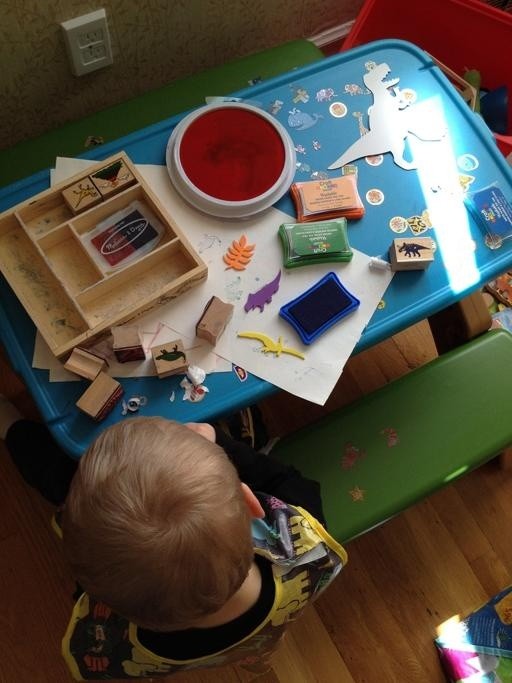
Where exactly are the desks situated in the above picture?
[1,40,512,473]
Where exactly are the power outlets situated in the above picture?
[61,9,115,78]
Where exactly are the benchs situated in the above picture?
[2,39,327,178]
[264,329,511,544]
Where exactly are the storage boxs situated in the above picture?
[334,1,512,157]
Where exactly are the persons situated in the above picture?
[0,385,350,681]
